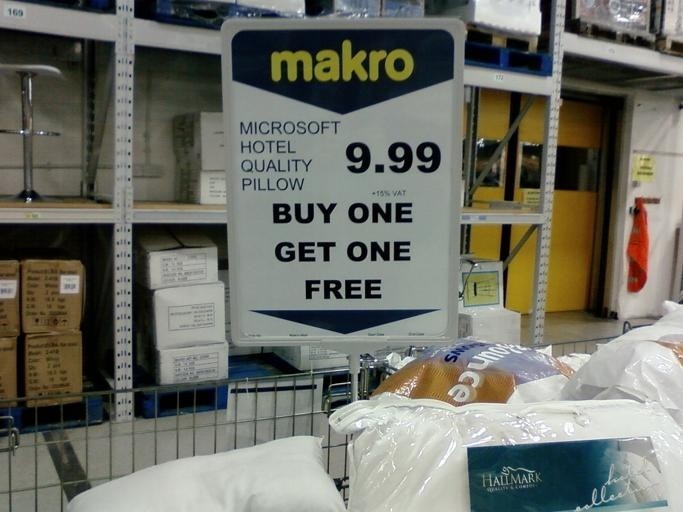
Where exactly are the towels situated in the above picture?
[628,197,651,290]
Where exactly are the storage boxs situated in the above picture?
[1,257,83,411]
[168,111,227,206]
[134,228,230,384]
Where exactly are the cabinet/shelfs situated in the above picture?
[0,0,571,433]
